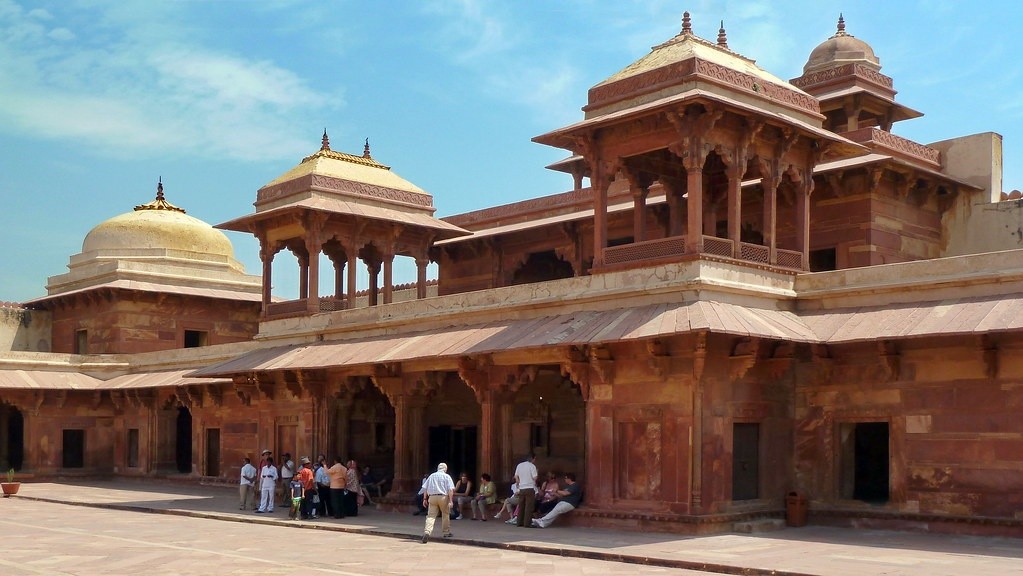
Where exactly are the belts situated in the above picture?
[284,478,288,479]
[433,494,442,495]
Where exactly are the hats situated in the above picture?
[301,458,310,464]
[262,449,272,456]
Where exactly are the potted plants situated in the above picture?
[1,468,21,494]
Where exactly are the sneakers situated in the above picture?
[532,518,544,528]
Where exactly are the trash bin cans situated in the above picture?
[786,488,807,527]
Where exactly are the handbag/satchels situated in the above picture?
[313,489,320,503]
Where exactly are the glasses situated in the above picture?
[282,458,286,459]
[550,477,556,480]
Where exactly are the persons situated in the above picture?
[421,464,456,544]
[239,450,386,523]
[412,452,583,529]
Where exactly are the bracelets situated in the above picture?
[481,494,483,496]
[548,500,549,502]
[424,499,427,501]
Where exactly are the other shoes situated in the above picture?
[239,508,243,510]
[456,514,464,519]
[255,511,264,513]
[279,505,291,507]
[509,517,518,524]
[288,517,292,519]
[471,518,478,520]
[308,513,313,519]
[269,510,273,513]
[505,519,514,523]
[301,514,307,519]
[295,518,299,520]
[494,513,501,518]
[443,533,453,537]
[422,533,429,543]
[480,518,487,521]
